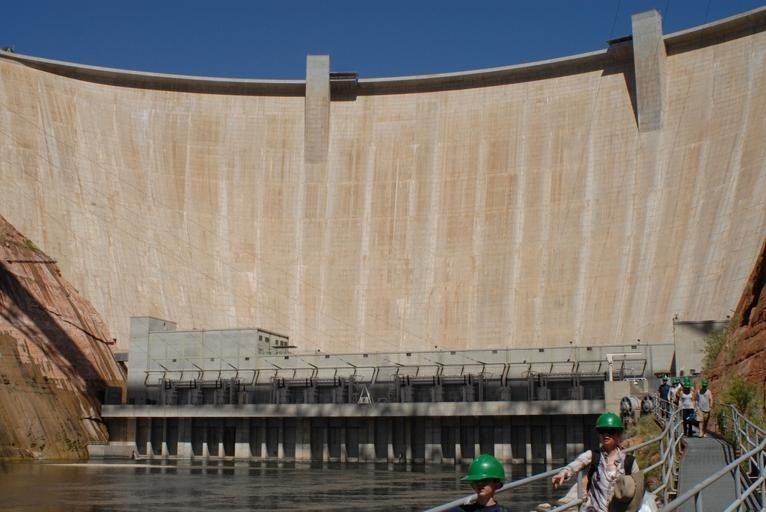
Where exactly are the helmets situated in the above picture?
[662,374,708,389]
[597,411,626,431]
[460,455,505,488]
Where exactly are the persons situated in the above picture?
[660,376,713,437]
[450,454,513,512]
[551,412,639,512]
